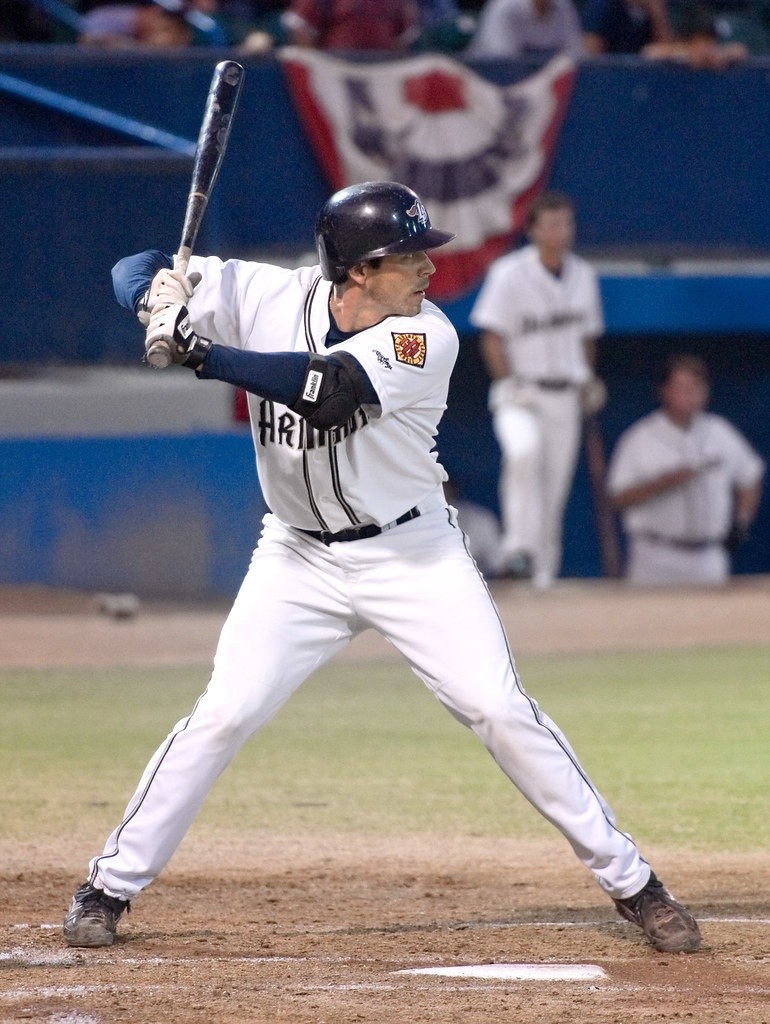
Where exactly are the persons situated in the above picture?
[603,354,764,583]
[65,181,702,954]
[467,193,605,583]
[466,0,746,69]
[77,0,228,47]
[287,0,420,50]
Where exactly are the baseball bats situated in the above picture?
[146,60,246,369]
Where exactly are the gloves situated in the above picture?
[138,269,213,377]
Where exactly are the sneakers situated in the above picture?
[611,869,704,951]
[63,881,127,948]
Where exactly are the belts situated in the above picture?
[301,506,420,548]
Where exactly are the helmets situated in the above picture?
[314,180,457,281]
